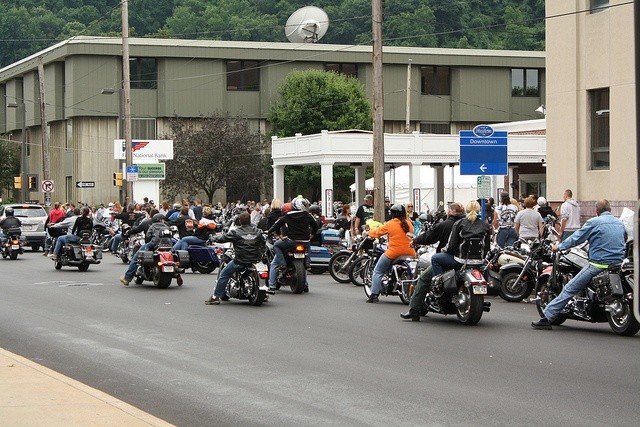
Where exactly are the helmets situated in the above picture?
[309,204,320,212]
[153,213,166,222]
[290,195,311,210]
[4,208,14,217]
[281,203,293,213]
[390,204,406,219]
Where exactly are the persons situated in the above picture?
[169,205,180,221]
[559,189,580,239]
[49,202,65,246]
[48,209,83,260]
[446,201,455,207]
[66,203,75,216]
[97,204,106,222]
[144,197,148,203]
[111,202,124,221]
[252,201,255,211]
[401,203,466,321]
[510,198,524,210]
[159,202,171,217]
[274,198,318,279]
[226,202,235,221]
[103,202,114,225]
[166,204,180,218]
[517,193,528,209]
[181,199,196,220]
[263,199,269,208]
[236,200,240,208]
[173,207,216,251]
[110,204,134,253]
[365,204,416,303]
[265,203,292,293]
[431,200,490,293]
[135,203,141,210]
[205,212,266,305]
[247,201,251,209]
[209,203,213,208]
[78,201,82,207]
[62,208,94,256]
[491,192,518,248]
[149,200,156,208]
[268,198,283,227]
[515,197,545,253]
[385,196,391,215]
[256,202,263,214]
[257,209,270,230]
[310,205,323,246]
[144,213,172,250]
[43,216,53,257]
[0,206,23,250]
[531,198,628,329]
[61,204,65,209]
[144,204,150,210]
[2,208,22,255]
[65,203,70,209]
[216,202,223,208]
[119,209,159,286]
[486,197,494,224]
[406,204,419,221]
[355,195,374,235]
[537,197,557,219]
[167,207,194,240]
[81,203,84,212]
[335,205,352,230]
[195,200,203,220]
[134,211,141,253]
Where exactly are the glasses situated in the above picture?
[385,200,390,203]
[408,206,413,207]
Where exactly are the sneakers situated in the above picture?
[48,253,57,259]
[205,295,220,305]
[120,278,129,286]
[177,273,182,286]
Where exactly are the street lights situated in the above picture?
[7,102,25,203]
[102,88,127,206]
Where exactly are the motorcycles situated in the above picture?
[91,218,131,263]
[48,223,103,271]
[535,234,640,337]
[364,234,419,304]
[0,227,24,259]
[124,230,189,289]
[406,232,491,325]
[263,231,309,294]
[308,229,375,287]
[207,234,275,306]
[487,202,564,302]
[171,233,223,273]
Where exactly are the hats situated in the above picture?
[537,197,546,205]
[364,195,373,200]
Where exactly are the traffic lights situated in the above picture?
[12,175,22,189]
[113,173,123,186]
[28,176,37,189]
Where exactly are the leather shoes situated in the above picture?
[531,317,552,330]
[400,308,420,321]
[367,295,378,303]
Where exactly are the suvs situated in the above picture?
[1,201,51,253]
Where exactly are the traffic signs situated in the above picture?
[76,181,95,187]
[460,125,508,175]
